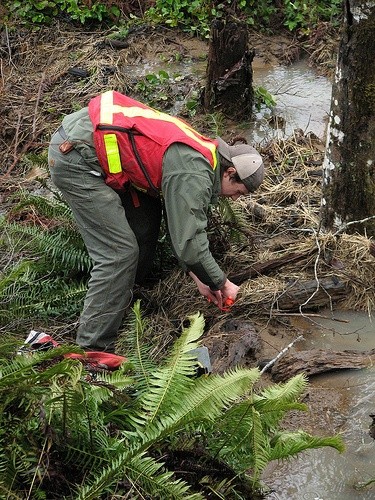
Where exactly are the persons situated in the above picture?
[47,89,265,354]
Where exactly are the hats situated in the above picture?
[215,134,265,192]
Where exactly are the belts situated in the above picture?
[58,126,67,140]
